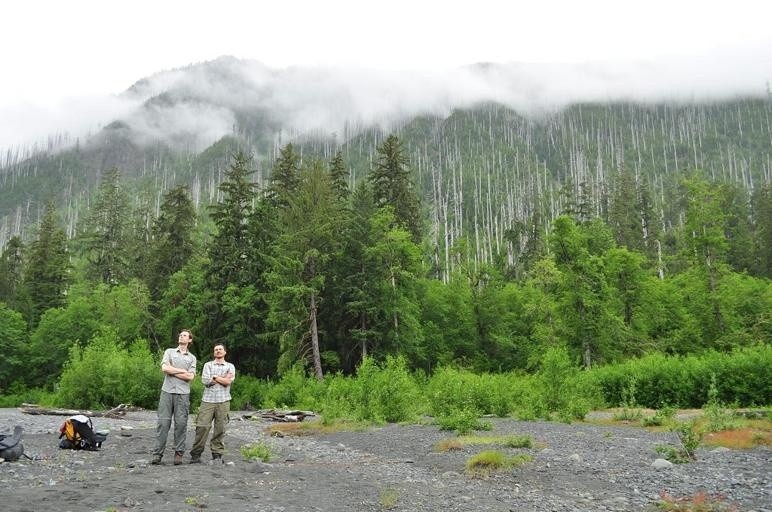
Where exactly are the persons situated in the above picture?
[189,341,235,464]
[153,327,197,465]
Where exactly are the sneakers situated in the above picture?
[173,452,183,465]
[213,455,224,463]
[152,453,163,464]
[190,455,200,464]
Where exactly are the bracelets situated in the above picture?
[213,374,217,380]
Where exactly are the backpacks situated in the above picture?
[0,427,33,463]
